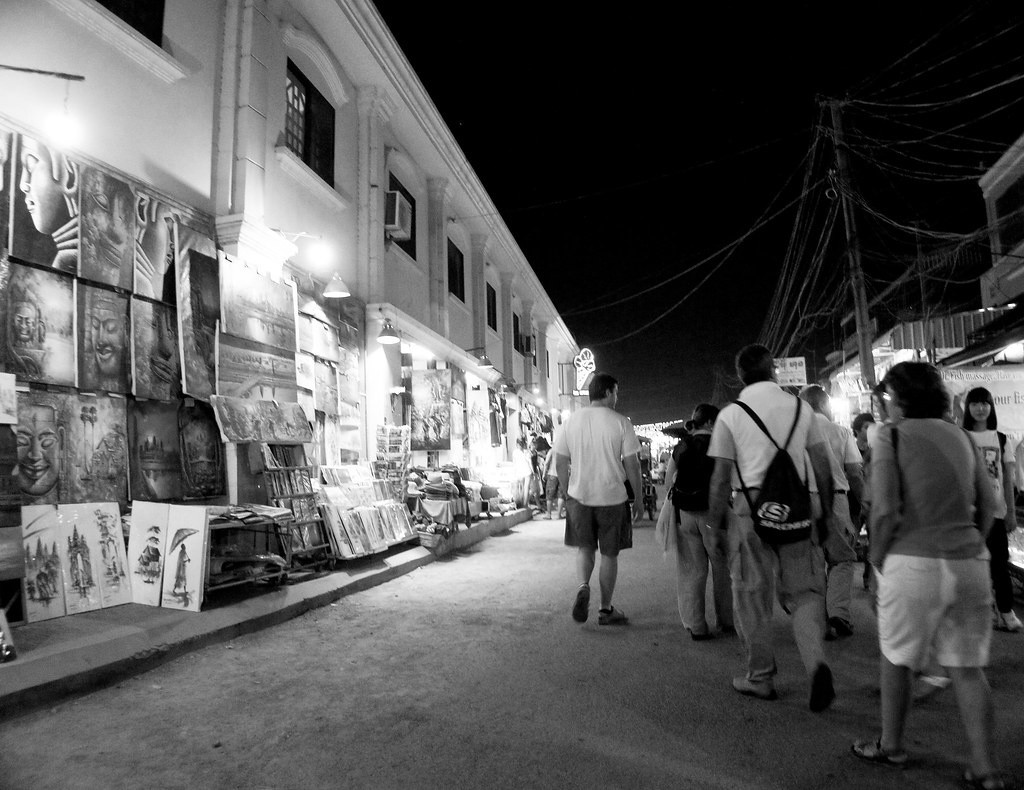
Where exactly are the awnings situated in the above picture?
[823,303,1024,380]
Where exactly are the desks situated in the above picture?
[417,497,469,535]
[204,518,294,601]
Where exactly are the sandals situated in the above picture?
[961,770,1008,790]
[850,740,913,770]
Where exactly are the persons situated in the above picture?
[647,458,670,483]
[850,361,1009,790]
[794,383,865,641]
[961,385,1024,632]
[846,413,877,592]
[510,439,567,523]
[665,401,737,642]
[703,343,836,713]
[554,372,646,626]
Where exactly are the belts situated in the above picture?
[834,489,847,493]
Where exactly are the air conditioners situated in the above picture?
[385,191,412,241]
[525,335,536,357]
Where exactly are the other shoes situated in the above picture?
[691,631,708,640]
[733,677,775,700]
[716,622,734,632]
[809,662,834,712]
[994,614,999,628]
[828,617,853,635]
[572,584,591,622]
[825,621,839,640]
[595,607,624,623]
[1001,610,1022,631]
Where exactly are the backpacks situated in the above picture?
[728,396,812,545]
[671,434,715,511]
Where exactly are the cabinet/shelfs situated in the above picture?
[373,423,411,506]
[259,443,336,576]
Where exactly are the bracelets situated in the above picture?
[867,553,881,566]
[705,524,718,536]
[1007,509,1017,513]
[634,498,644,504]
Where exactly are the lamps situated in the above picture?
[478,348,493,369]
[323,272,350,299]
[376,318,401,344]
